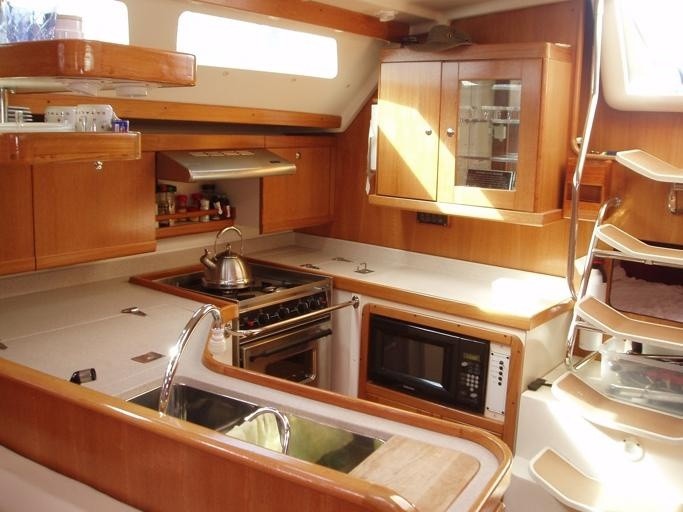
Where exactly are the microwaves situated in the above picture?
[365,311,491,418]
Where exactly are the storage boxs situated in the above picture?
[600,336,683,418]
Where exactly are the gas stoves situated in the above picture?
[154,261,320,304]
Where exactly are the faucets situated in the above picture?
[156,302,227,411]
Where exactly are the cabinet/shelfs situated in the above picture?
[157,150,297,249]
[0,39,196,167]
[260,148,334,234]
[563,197,682,419]
[377,60,543,214]
[0,145,38,274]
[31,146,157,272]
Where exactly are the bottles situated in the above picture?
[156,182,232,226]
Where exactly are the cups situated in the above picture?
[44,104,121,133]
[54,14,84,39]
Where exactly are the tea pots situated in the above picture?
[198,225,254,293]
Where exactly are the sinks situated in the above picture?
[223,404,391,476]
[114,374,260,431]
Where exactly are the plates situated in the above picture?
[1,122,77,133]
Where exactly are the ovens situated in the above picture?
[231,284,336,389]
